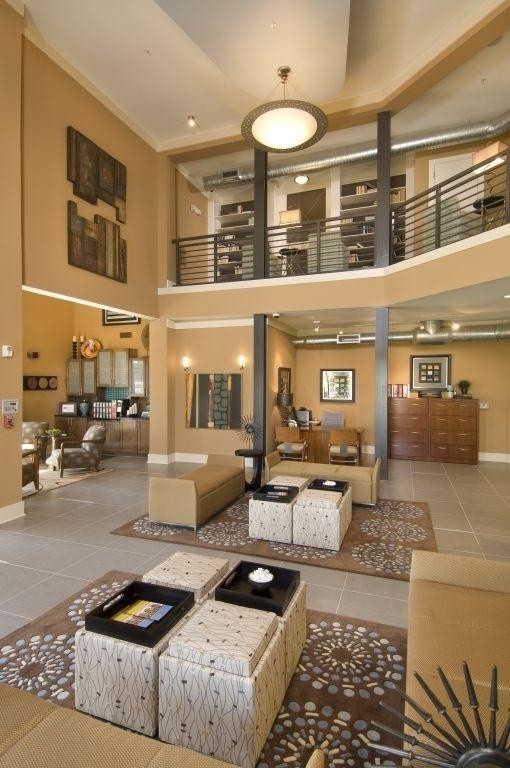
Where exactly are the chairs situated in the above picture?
[275,424,361,466]
[23,421,107,492]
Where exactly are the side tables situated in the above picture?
[235,448,265,490]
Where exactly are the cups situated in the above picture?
[447,385,454,392]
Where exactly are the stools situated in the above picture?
[280,249,300,276]
[474,197,505,230]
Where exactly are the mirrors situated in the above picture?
[184,372,243,433]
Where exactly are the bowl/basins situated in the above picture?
[244,572,275,591]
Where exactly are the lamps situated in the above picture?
[241,67,327,153]
[472,141,508,172]
[278,204,303,229]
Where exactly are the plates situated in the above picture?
[27,377,38,388]
[39,378,48,389]
[49,377,58,389]
[80,338,101,358]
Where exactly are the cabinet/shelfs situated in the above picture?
[217,251,242,280]
[53,414,149,456]
[347,246,374,266]
[428,396,479,466]
[386,397,429,465]
[65,347,149,397]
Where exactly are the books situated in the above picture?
[341,218,354,224]
[357,185,367,194]
[346,241,364,248]
[248,217,254,225]
[218,256,229,263]
[216,246,240,253]
[347,253,358,262]
[389,190,406,203]
[387,384,409,399]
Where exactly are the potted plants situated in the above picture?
[458,380,470,395]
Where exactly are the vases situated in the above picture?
[79,402,90,417]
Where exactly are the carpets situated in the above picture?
[1,566,407,768]
[22,463,114,499]
[110,493,437,581]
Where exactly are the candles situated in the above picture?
[80,335,85,342]
[72,335,77,342]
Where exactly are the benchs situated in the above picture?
[1,681,240,768]
[264,450,382,506]
[147,455,245,532]
[403,548,510,767]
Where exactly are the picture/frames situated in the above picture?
[319,368,355,403]
[102,309,141,326]
[409,354,451,390]
[278,367,291,394]
